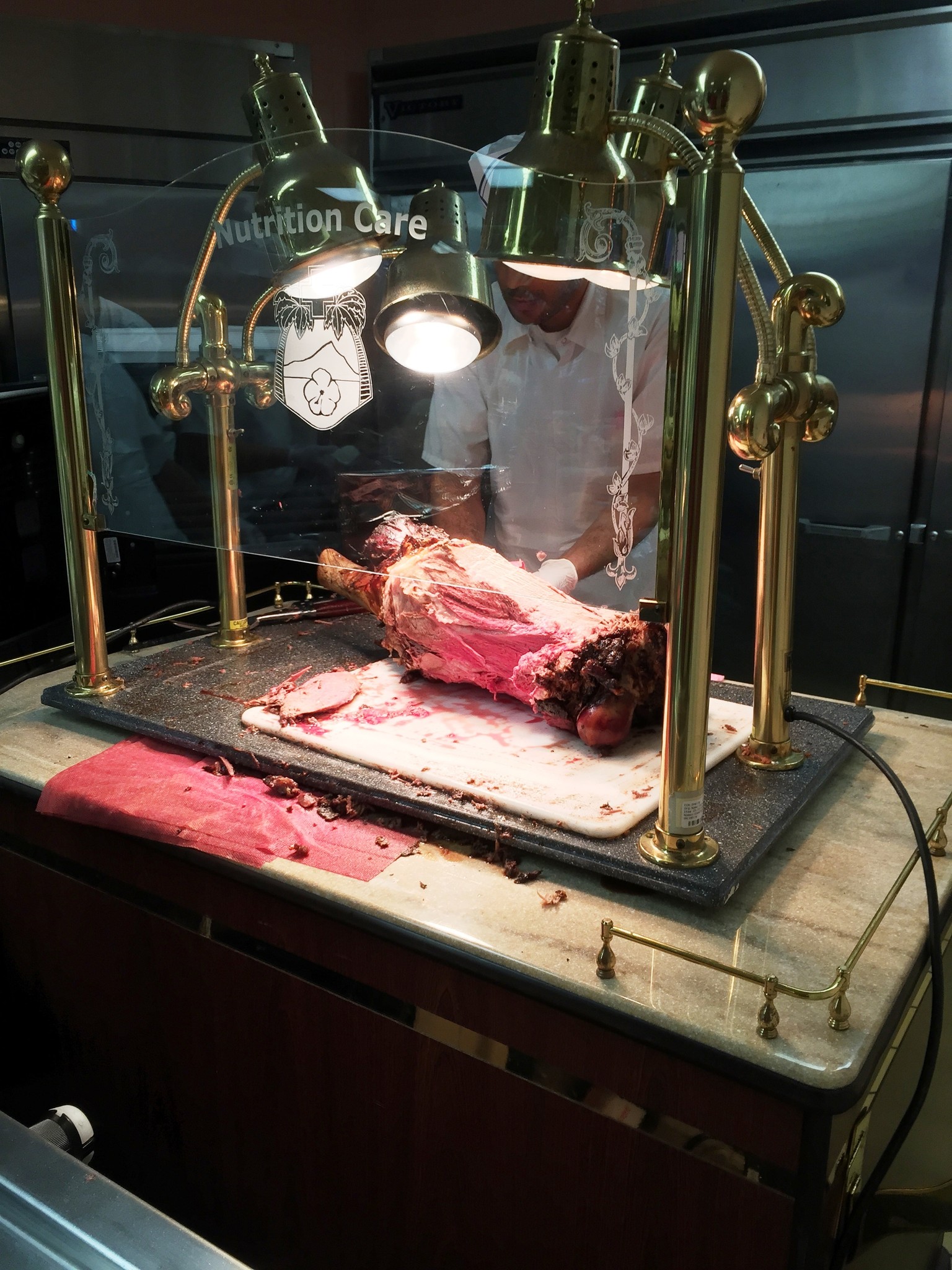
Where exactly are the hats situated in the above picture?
[467,131,526,219]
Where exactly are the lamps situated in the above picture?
[151,50,503,650]
[485,1,847,773]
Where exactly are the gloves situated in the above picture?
[531,558,579,593]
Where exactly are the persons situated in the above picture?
[423,136,669,610]
[48,278,181,547]
